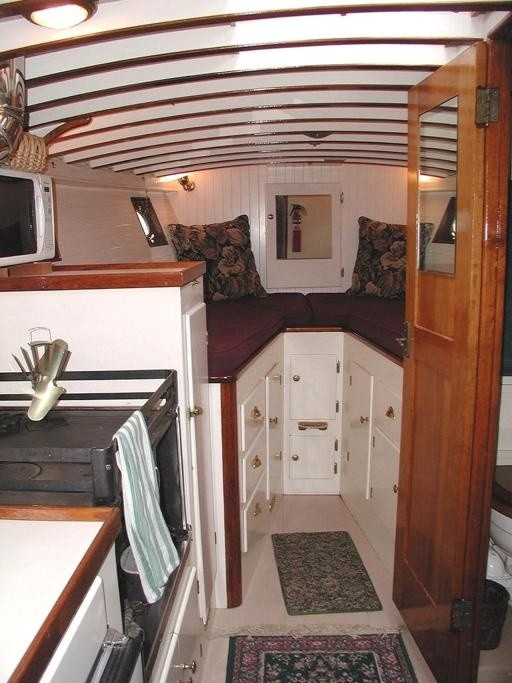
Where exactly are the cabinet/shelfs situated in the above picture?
[284,331,340,496]
[0,260,218,624]
[341,333,403,579]
[208,328,285,609]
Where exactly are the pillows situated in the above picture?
[345,214,435,304]
[170,214,267,302]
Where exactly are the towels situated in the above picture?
[112,410,182,604]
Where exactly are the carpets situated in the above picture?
[225,632,419,683]
[272,531,383,615]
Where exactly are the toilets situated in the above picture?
[486,375,512,609]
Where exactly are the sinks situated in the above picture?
[1,520,103,681]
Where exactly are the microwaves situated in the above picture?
[0,167,58,267]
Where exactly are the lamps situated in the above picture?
[18,0,98,30]
[177,174,195,192]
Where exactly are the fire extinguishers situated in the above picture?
[289,204,305,252]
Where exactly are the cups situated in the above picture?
[477,578,510,651]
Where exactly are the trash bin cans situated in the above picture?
[480,580,509,651]
